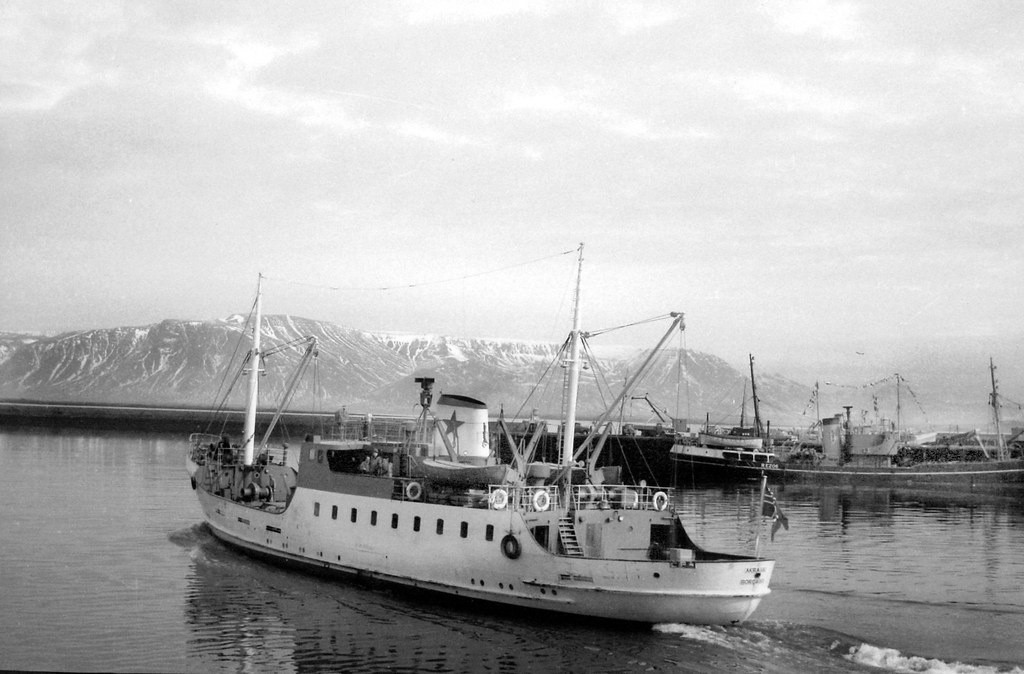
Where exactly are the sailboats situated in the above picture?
[184,242,776,626]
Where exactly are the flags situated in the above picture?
[761,485,788,542]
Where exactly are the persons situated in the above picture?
[358,448,393,477]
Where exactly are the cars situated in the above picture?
[558,422,590,435]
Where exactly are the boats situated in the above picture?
[669,427,779,470]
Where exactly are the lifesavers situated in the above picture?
[653,491,669,512]
[533,490,549,511]
[406,482,421,499]
[492,489,507,508]
[500,534,521,559]
